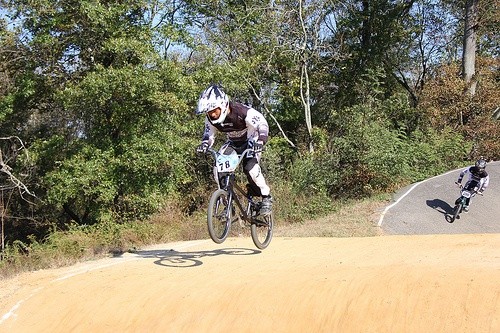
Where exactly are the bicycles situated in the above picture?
[451,180,484,225]
[193,144,275,250]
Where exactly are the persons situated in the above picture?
[191,84,273,218]
[456,158,489,213]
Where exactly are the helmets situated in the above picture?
[475,159,486,174]
[197,85,229,124]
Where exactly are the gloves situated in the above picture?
[457,181,461,187]
[252,139,264,152]
[197,142,209,154]
[479,188,483,194]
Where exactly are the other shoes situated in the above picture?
[259,194,274,217]
[464,205,469,211]
[220,205,235,218]
[455,197,462,204]
